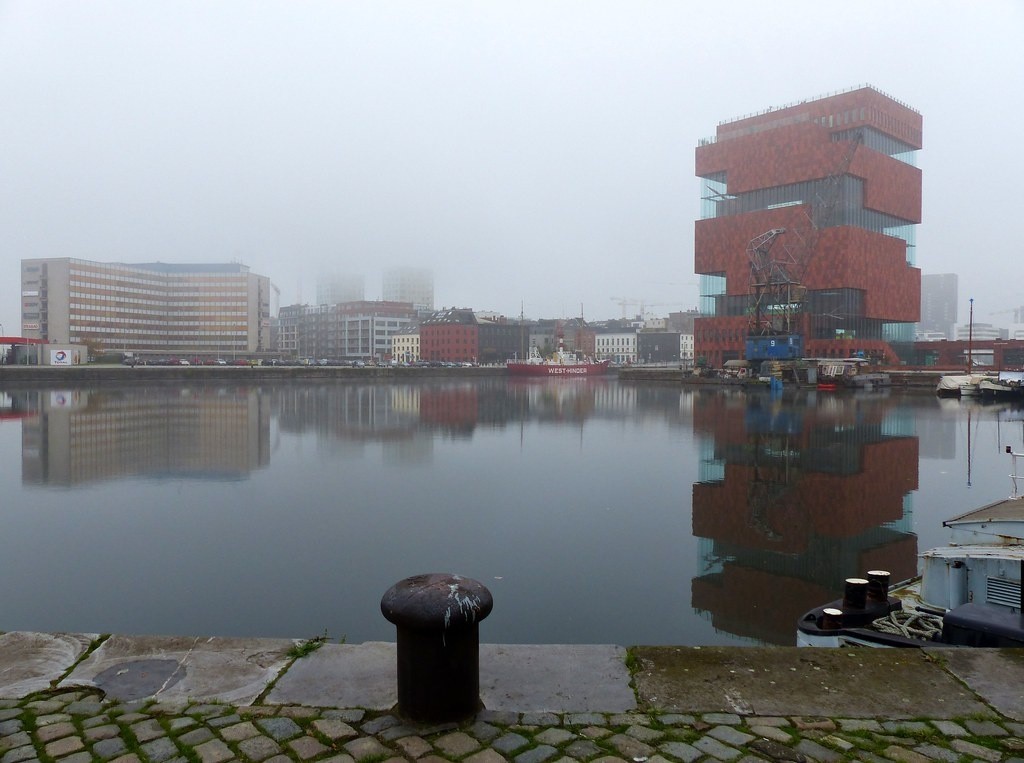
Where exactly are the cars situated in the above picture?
[122,358,480,368]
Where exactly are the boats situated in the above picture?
[505,317,611,377]
[842,368,893,388]
[795,445,1024,648]
[938,297,1024,399]
[684,367,748,386]
